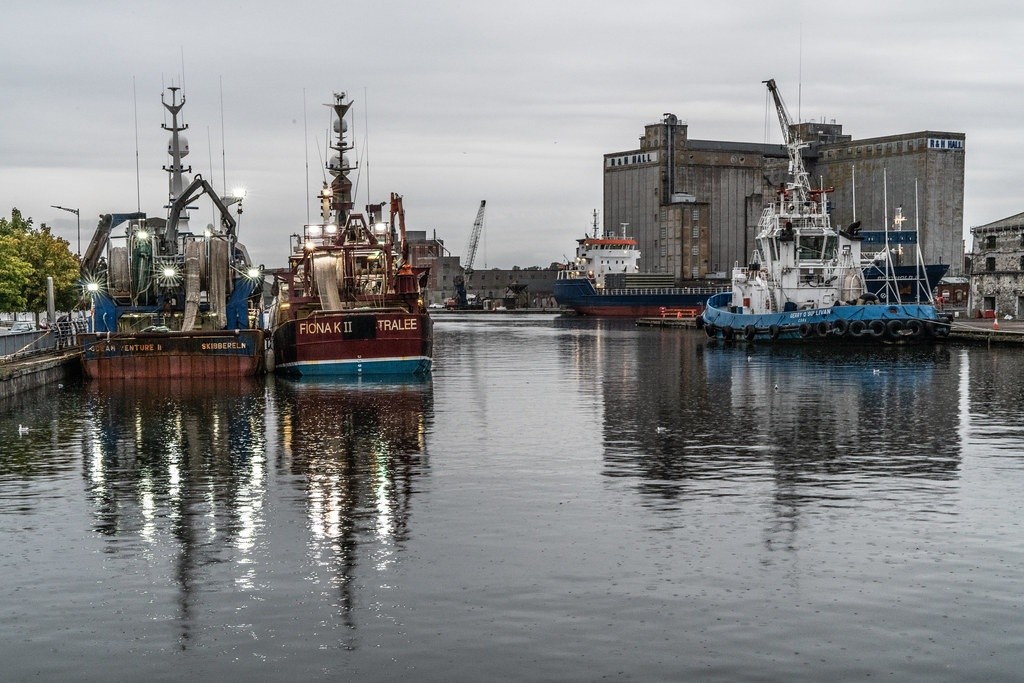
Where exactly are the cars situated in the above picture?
[39,317,47,329]
[8,322,33,333]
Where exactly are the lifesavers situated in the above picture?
[868,320,887,337]
[705,324,714,337]
[849,320,867,338]
[816,320,831,338]
[906,320,923,338]
[768,324,779,339]
[743,324,755,340]
[696,315,702,329]
[832,318,849,338]
[798,323,812,337]
[887,320,905,338]
[758,270,768,279]
[721,326,732,342]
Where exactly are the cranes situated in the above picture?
[445,199,487,310]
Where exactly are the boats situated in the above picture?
[555,208,732,316]
[695,78,952,344]
[80,48,272,380]
[265,86,434,378]
